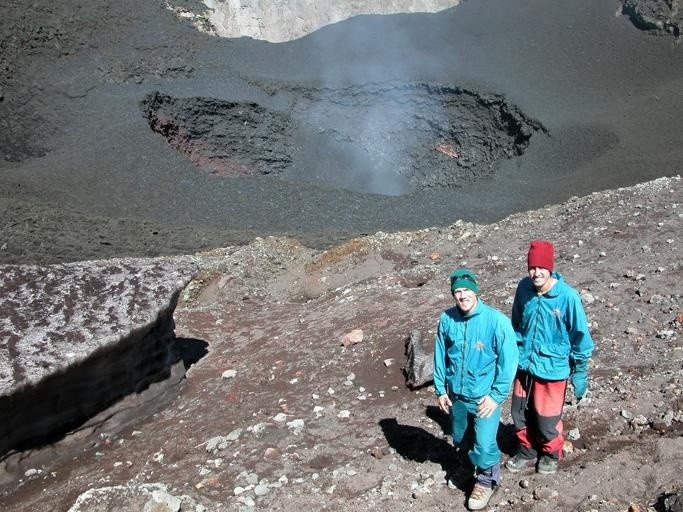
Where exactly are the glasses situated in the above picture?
[452,274,477,284]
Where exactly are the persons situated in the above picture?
[430,267,519,511]
[504,240,596,475]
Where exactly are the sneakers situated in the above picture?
[505,455,558,472]
[441,441,494,509]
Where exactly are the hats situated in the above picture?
[450,268,478,294]
[528,241,553,273]
